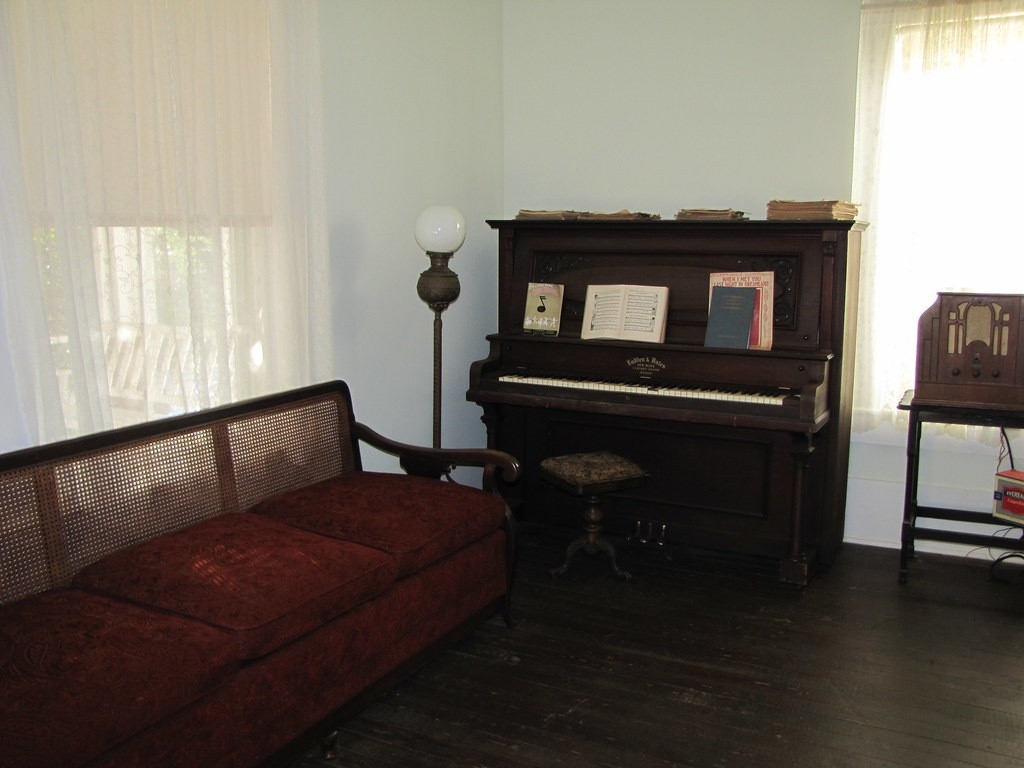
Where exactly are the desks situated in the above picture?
[896,388,1024,588]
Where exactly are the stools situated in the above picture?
[539,447,650,589]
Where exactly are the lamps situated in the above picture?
[412,205,469,265]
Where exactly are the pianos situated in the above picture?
[463,216,871,588]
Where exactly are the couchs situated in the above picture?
[0,380,525,768]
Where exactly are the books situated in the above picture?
[704,271,773,350]
[578,284,669,344]
[523,282,564,338]
